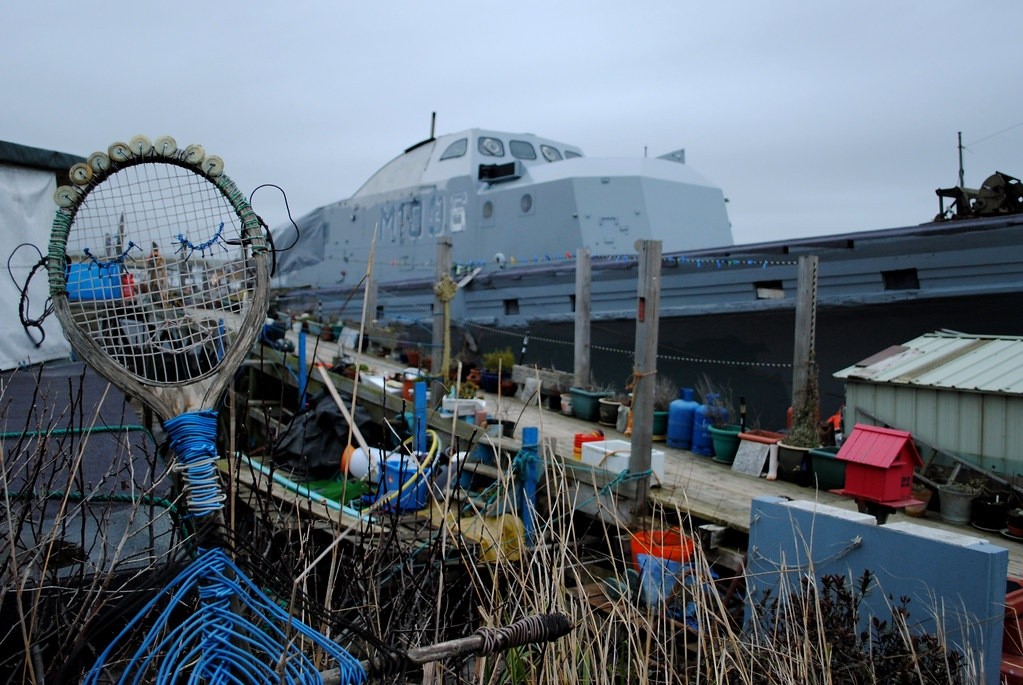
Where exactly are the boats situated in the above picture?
[242,110,1021,436]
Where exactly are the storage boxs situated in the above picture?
[380,460,431,511]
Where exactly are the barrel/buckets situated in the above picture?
[632,532,692,574]
[574,433,604,461]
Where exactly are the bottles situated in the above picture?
[341,445,417,484]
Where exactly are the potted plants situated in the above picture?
[938,482,1023,540]
[450,346,517,396]
[278,309,432,370]
[334,358,370,382]
[511,357,633,431]
[654,376,678,436]
[904,483,933,515]
[692,371,823,486]
[442,380,485,411]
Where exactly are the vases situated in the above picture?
[808,445,848,490]
